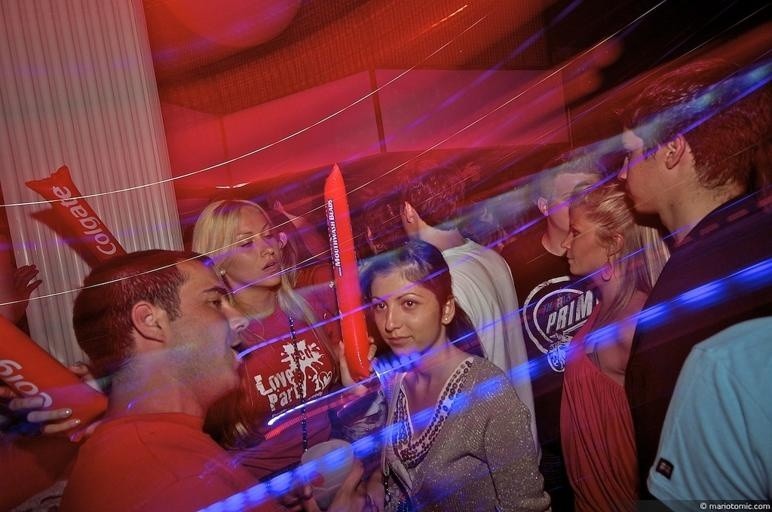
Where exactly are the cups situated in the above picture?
[298,440,357,510]
